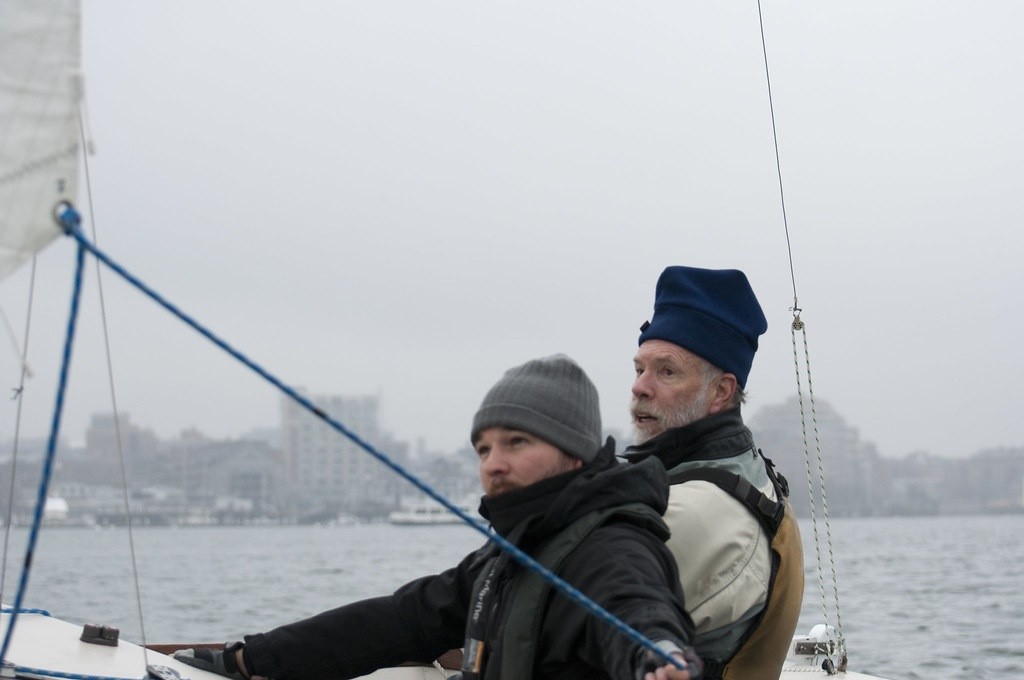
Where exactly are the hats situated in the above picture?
[639,265,768,390]
[470,352,601,467]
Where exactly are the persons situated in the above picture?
[613,265,805,680]
[167,354,704,680]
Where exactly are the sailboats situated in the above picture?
[0,2,900,680]
